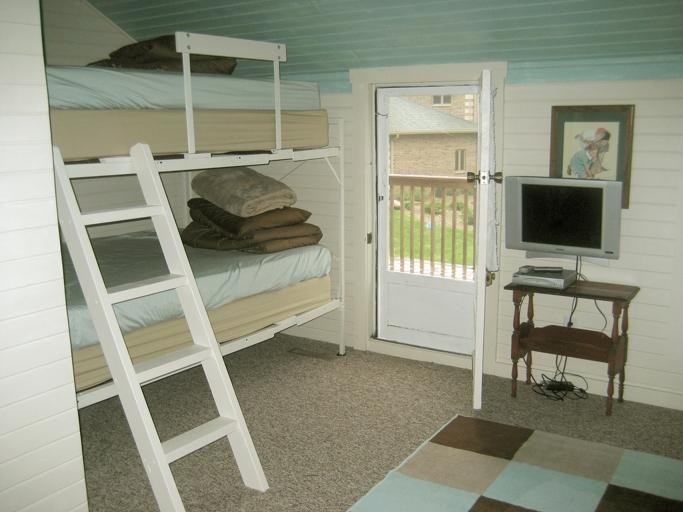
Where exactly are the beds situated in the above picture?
[46,32,346,512]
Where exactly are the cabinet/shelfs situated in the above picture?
[504,280,640,415]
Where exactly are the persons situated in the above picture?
[573,127,606,145]
[590,131,610,173]
[564,143,598,180]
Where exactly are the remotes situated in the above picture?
[519,264,534,275]
[534,266,564,273]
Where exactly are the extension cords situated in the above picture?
[542,381,575,392]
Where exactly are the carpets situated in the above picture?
[345,415,683,512]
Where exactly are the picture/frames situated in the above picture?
[550,105,636,209]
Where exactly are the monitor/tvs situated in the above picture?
[503,175,623,260]
